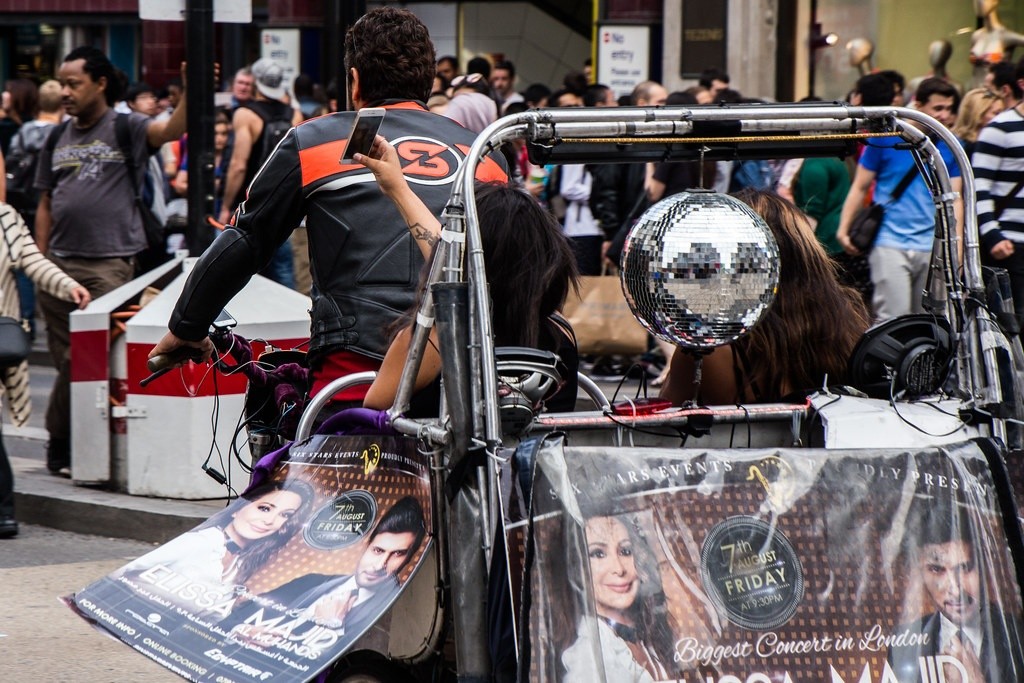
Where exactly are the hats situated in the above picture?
[252,57,285,99]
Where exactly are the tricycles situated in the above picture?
[134,104,1022,683]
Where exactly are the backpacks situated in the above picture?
[5,129,43,194]
[42,113,169,229]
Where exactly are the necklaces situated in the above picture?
[222,530,243,557]
[596,613,644,644]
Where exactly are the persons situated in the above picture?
[216,495,426,665]
[845,35,877,78]
[352,132,578,440]
[32,45,220,486]
[106,474,314,630]
[151,6,515,440]
[429,46,1024,386]
[658,187,873,413]
[884,500,1020,682]
[969,0,1023,78]
[0,154,92,541]
[542,495,684,682]
[0,47,343,314]
[908,39,965,98]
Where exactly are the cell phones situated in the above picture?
[212,307,238,330]
[339,107,388,167]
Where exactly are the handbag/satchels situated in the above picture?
[0,317,31,359]
[849,204,885,255]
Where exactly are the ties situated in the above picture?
[950,630,974,650]
[349,588,359,601]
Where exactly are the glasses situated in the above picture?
[134,94,158,103]
[451,72,489,87]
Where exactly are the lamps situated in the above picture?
[813,32,839,50]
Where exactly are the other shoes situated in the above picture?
[51,466,71,479]
[0,516,18,537]
